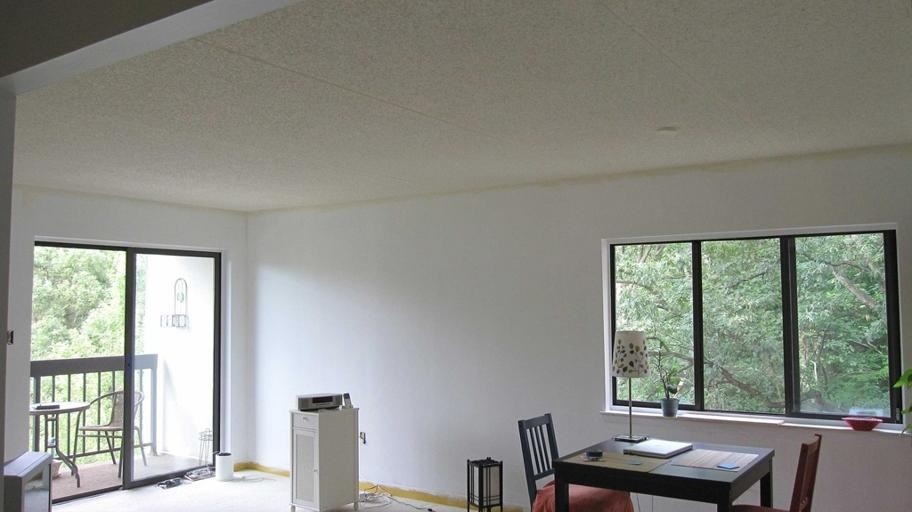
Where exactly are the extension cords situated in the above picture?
[360,493,375,501]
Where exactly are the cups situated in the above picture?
[585,450,602,462]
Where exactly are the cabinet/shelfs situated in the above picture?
[290,408,359,512]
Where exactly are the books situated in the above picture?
[35,402,60,409]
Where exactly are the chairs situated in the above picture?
[72,390,146,477]
[518,413,629,512]
[733,432,822,512]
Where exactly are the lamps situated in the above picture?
[610,331,648,443]
[467,457,502,512]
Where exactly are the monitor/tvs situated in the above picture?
[0,451,52,512]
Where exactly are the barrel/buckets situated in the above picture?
[215,453,234,481]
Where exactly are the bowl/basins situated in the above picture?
[840,416,883,431]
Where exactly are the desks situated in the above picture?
[29,402,90,488]
[553,434,775,512]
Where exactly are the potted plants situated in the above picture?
[652,341,680,417]
